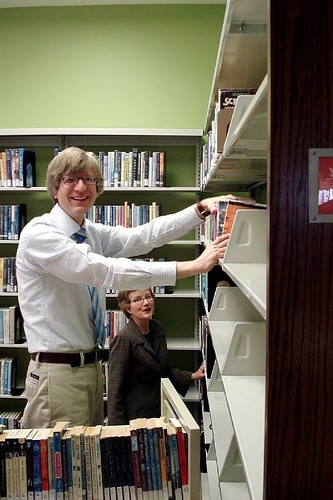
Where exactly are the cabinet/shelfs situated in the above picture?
[0,128,203,401]
[202,0,333,499]
[160,377,202,500]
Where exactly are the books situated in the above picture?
[199,264,238,412]
[0,148,36,429]
[199,88,259,242]
[54,149,164,396]
[199,415,208,474]
[0,418,188,500]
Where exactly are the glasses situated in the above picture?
[128,294,154,303]
[58,174,100,185]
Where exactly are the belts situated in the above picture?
[31,348,102,367]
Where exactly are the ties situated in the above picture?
[72,227,107,351]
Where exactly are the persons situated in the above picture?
[16,145,240,429]
[107,287,206,426]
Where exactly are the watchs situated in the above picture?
[197,201,209,217]
